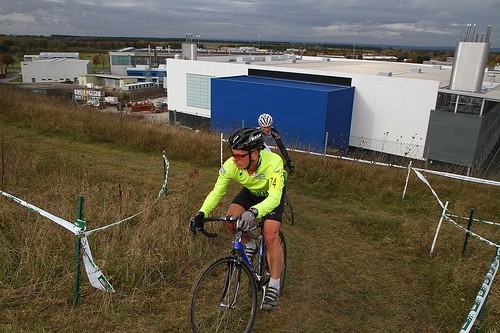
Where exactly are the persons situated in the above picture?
[189,128,286,311]
[255,114,294,173]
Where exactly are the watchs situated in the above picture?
[247,208,258,218]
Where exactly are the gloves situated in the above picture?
[236,210,257,233]
[286,160,295,174]
[189,212,205,233]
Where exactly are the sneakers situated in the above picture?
[262,283,282,311]
[243,241,258,264]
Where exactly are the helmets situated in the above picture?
[228,126,266,153]
[258,113,274,127]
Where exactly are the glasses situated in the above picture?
[230,149,257,161]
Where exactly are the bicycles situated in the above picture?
[187,212,288,333]
[282,163,294,225]
